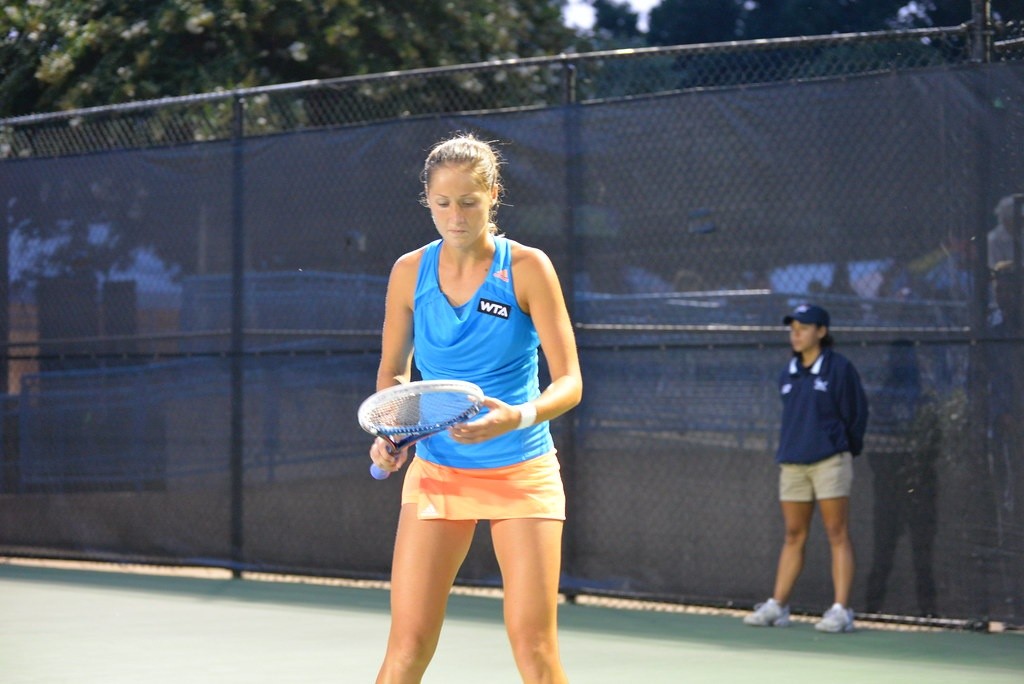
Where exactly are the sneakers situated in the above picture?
[744,598,790,627]
[815,602,855,633]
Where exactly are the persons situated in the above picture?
[744,305,867,632]
[370,137,582,684]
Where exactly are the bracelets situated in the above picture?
[513,402,536,430]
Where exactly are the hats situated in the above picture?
[782,304,830,327]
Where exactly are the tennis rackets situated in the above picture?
[353,378,488,482]
